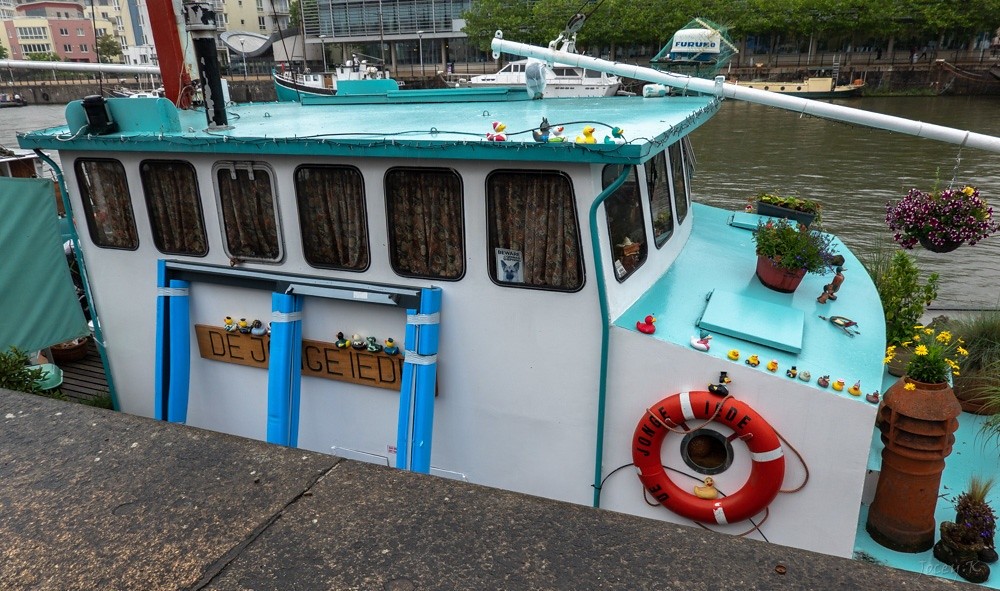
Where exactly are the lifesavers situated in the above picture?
[632,391,786,526]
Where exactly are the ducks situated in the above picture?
[636,315,657,334]
[866,390,882,404]
[533,118,550,142]
[486,121,508,142]
[604,127,626,144]
[729,349,740,360]
[847,380,863,396]
[799,370,811,381]
[548,126,568,142]
[708,372,731,397]
[576,125,597,144]
[223,316,271,337]
[335,332,400,355]
[818,375,830,388]
[691,329,713,351]
[832,379,845,390]
[787,366,798,379]
[746,354,760,367]
[766,359,778,372]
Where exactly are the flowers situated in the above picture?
[939,473,999,541]
[751,215,841,277]
[882,326,970,391]
[884,165,1000,250]
[743,188,827,232]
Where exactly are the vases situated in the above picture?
[920,238,965,253]
[755,256,807,294]
[757,202,814,228]
[50,338,89,362]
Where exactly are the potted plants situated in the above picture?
[877,249,941,378]
[923,294,1000,416]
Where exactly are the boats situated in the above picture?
[470,56,637,98]
[0,0,1000,591]
[441,74,483,88]
[100,74,205,105]
[933,57,1000,96]
[0,91,27,107]
[271,55,399,104]
[671,76,866,99]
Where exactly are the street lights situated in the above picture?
[318,35,329,72]
[239,39,249,80]
[150,46,155,65]
[416,30,426,76]
